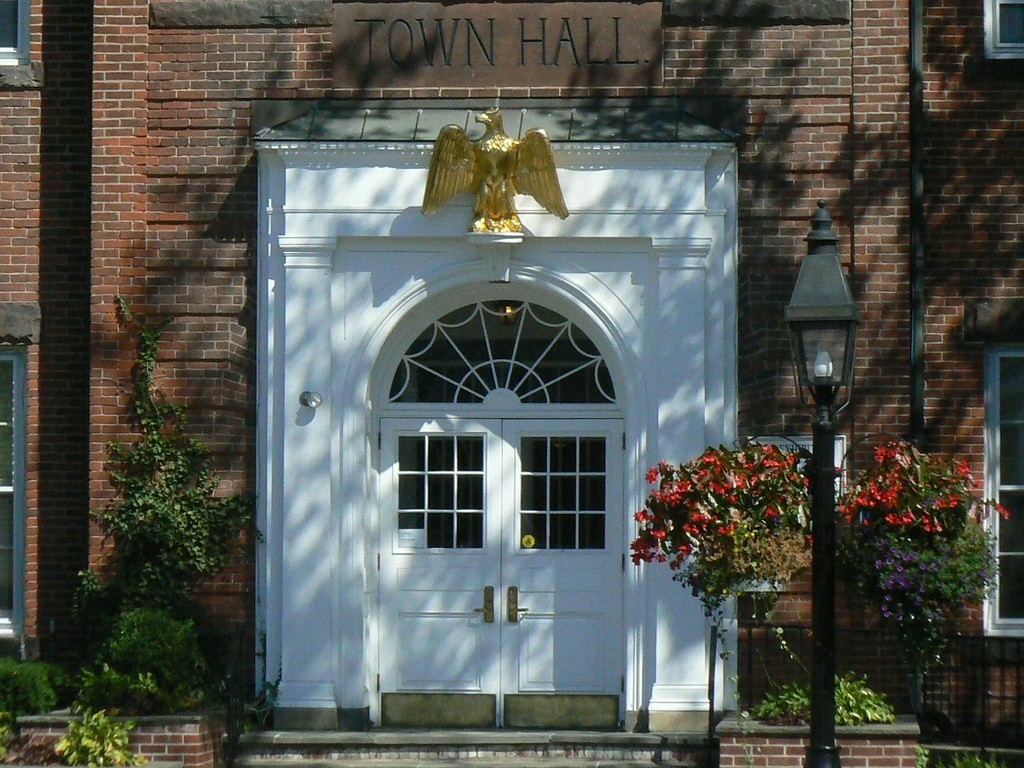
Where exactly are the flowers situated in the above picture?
[607,431,816,607]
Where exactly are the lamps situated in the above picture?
[493,300,523,327]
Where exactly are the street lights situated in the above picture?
[783,198,862,768]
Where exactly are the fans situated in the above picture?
[832,425,1007,670]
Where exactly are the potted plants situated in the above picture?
[716,666,921,768]
[13,608,223,768]
